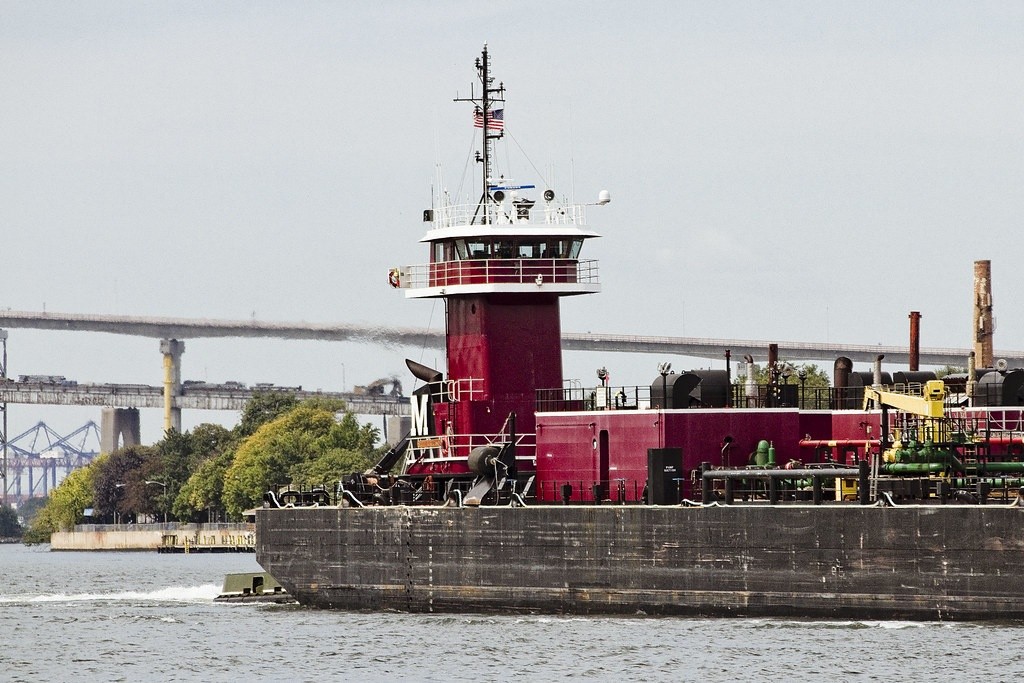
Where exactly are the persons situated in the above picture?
[514,254,523,275]
[443,421,454,458]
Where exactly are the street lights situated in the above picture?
[145,481,167,522]
[116,484,138,524]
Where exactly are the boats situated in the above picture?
[255,42,1024,619]
[213,592,295,605]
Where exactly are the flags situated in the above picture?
[473,109,504,131]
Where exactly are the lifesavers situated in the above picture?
[280,489,329,507]
[389,272,399,288]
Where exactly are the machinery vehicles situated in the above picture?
[352,376,402,399]
